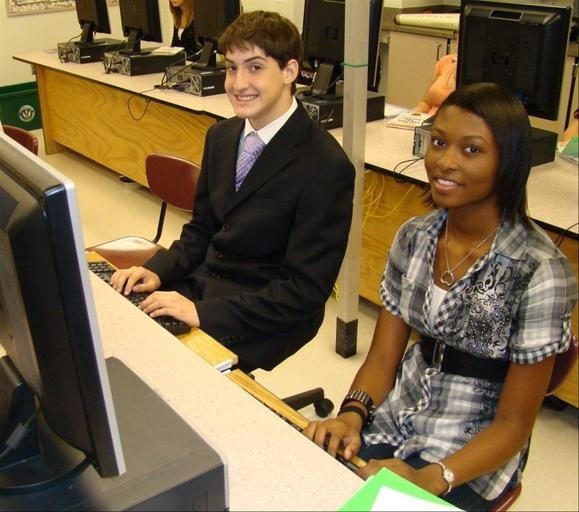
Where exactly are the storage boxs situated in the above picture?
[0,81,42,131]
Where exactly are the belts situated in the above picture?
[419,332,509,379]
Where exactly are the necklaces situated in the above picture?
[438,215,502,287]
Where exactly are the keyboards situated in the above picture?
[87,262,191,335]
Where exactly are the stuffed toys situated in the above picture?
[412,51,458,116]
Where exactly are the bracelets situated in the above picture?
[340,387,378,426]
[333,404,369,427]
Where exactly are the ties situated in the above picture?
[235,132,265,192]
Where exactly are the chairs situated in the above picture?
[0,125,38,156]
[484,325,579,512]
[85,153,202,269]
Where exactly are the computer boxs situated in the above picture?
[104,47,186,76]
[164,66,228,96]
[413,123,558,167]
[57,38,127,63]
[294,84,384,130]
[1,355,223,512]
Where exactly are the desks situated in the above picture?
[0,268,404,512]
[12,29,579,414]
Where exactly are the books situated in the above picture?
[386,112,432,129]
[150,45,185,57]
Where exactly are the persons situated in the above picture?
[559,107,579,145]
[106,10,359,381]
[299,80,579,512]
[168,1,205,64]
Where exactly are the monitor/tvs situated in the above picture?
[191,0,240,70]
[1,133,125,488]
[119,0,162,56]
[456,0,573,120]
[74,0,111,45]
[299,0,385,100]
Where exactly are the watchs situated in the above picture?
[433,460,456,497]
